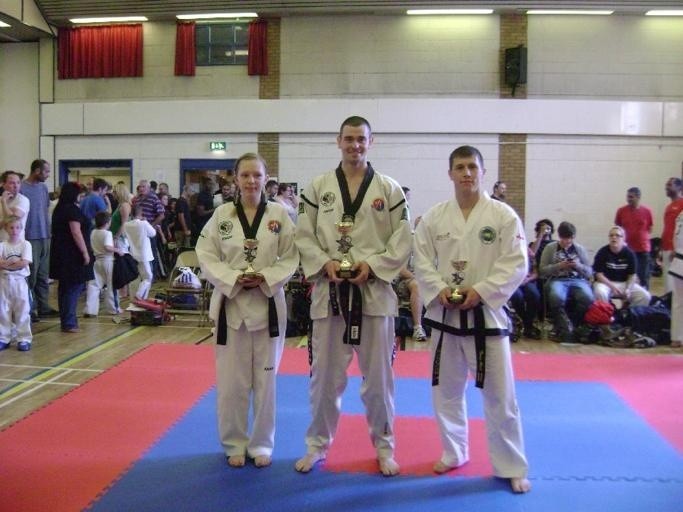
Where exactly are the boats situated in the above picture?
[505,47,527,85]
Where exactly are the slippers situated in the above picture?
[577,299,673,349]
[112,252,141,290]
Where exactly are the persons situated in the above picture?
[195,152,299,468]
[401,186,410,201]
[491,180,507,200]
[412,145,531,493]
[212,179,298,223]
[510,177,683,348]
[293,115,413,477]
[1,159,61,352]
[48,177,216,332]
[391,216,427,341]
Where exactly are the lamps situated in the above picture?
[163,251,207,326]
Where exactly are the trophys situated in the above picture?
[244,238,259,275]
[335,221,353,279]
[449,261,468,303]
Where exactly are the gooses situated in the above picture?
[449,261,468,303]
[335,221,353,279]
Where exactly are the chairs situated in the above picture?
[30,313,40,322]
[60,327,81,333]
[1,342,9,351]
[522,323,541,340]
[412,328,427,342]
[38,310,61,318]
[17,342,30,351]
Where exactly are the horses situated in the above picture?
[567,259,573,263]
